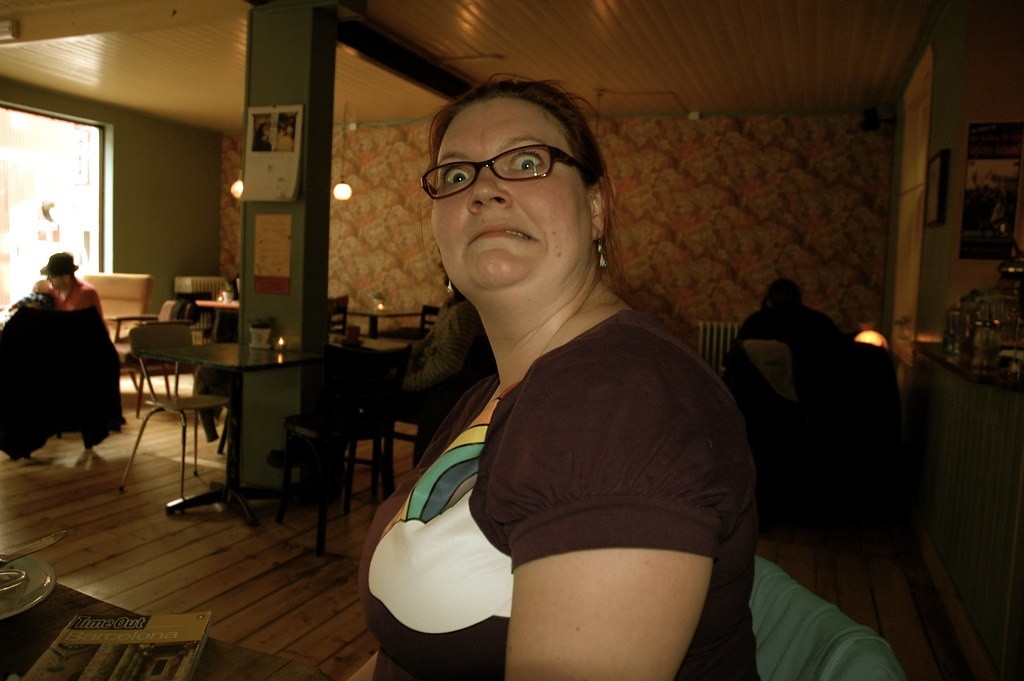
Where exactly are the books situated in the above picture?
[19,611,211,681]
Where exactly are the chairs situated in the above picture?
[277,340,415,561]
[106,299,185,418]
[56,273,159,439]
[119,320,231,497]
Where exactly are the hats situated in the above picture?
[40,253,80,275]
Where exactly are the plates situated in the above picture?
[341,339,364,347]
[0,556,57,620]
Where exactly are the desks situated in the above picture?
[328,335,415,500]
[141,343,303,529]
[346,307,422,339]
[0,582,330,681]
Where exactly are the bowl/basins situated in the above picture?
[0,570,26,607]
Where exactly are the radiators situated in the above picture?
[696,320,739,373]
[175,276,228,331]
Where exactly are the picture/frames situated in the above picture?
[926,149,952,227]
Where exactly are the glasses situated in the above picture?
[420,144,593,200]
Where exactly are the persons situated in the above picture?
[366,70,760,681]
[36,253,103,312]
[266,249,489,494]
[722,277,853,476]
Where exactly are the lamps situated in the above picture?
[230,110,244,199]
[333,104,353,201]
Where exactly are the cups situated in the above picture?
[1007,317,1024,385]
[347,326,360,343]
[945,294,1007,371]
